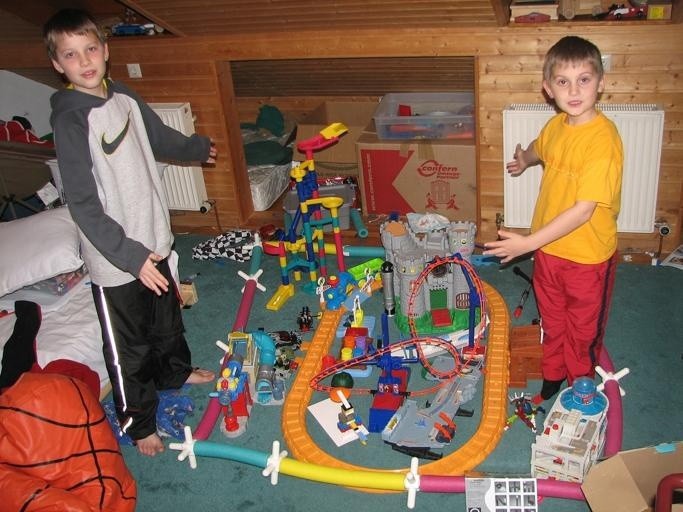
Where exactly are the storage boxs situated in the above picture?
[354,116,479,224]
[372,91,476,140]
[579,437,683,512]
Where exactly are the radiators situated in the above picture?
[144,101,215,217]
[498,103,666,236]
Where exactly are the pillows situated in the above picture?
[0,205,87,301]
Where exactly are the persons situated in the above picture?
[43,8,217,456]
[480,36,622,400]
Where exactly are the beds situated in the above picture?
[0,248,193,403]
